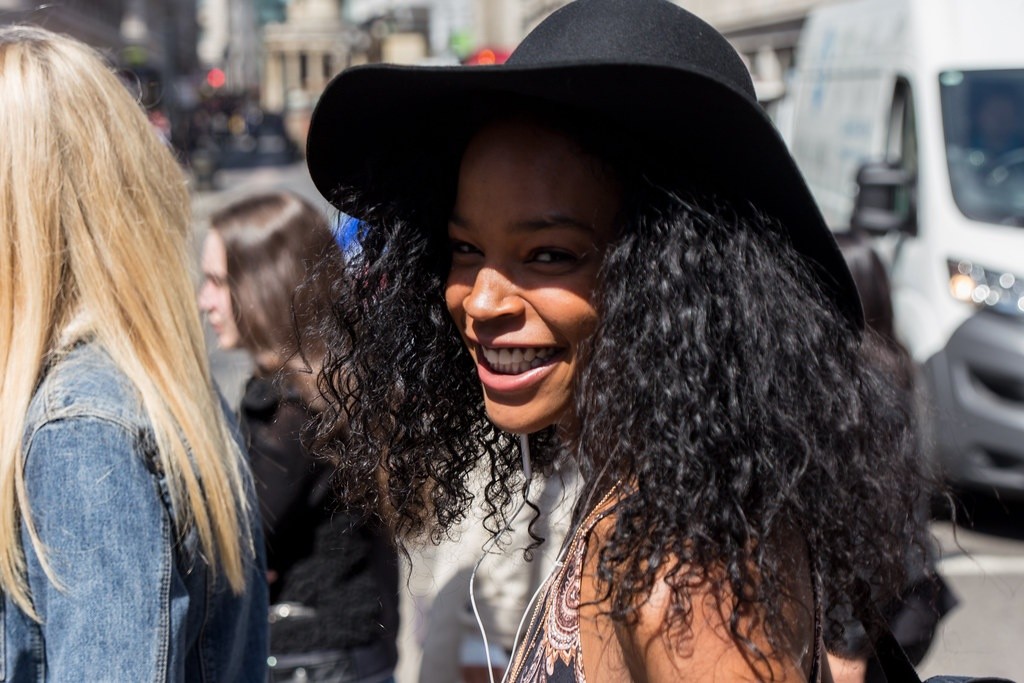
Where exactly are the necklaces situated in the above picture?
[503,472,628,683]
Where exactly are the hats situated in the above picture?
[306,0,865,334]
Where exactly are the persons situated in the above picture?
[199,181,398,683]
[262,1,1013,683]
[0,15,273,683]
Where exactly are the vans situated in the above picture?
[790,0,1024,520]
